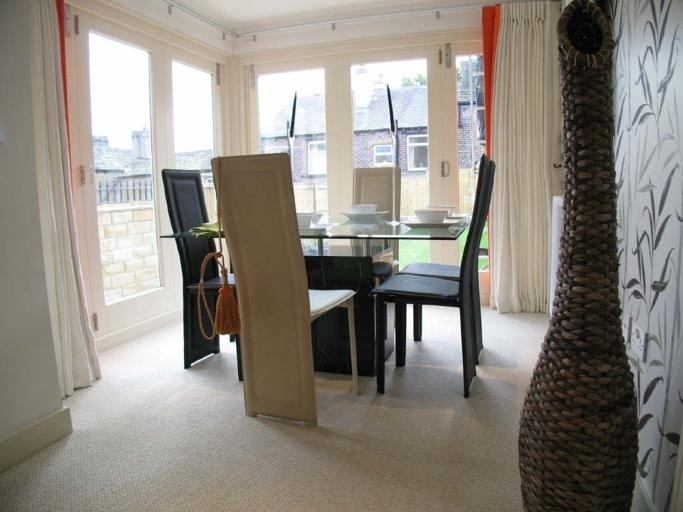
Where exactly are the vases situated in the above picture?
[518,0,639,512]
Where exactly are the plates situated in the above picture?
[343,211,391,220]
[406,220,459,228]
[309,223,334,231]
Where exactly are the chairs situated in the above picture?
[161,168,243,382]
[353,166,401,270]
[394,161,496,367]
[211,153,360,428]
[369,153,490,399]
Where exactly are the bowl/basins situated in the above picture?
[415,209,448,223]
[351,204,377,214]
[296,213,312,230]
